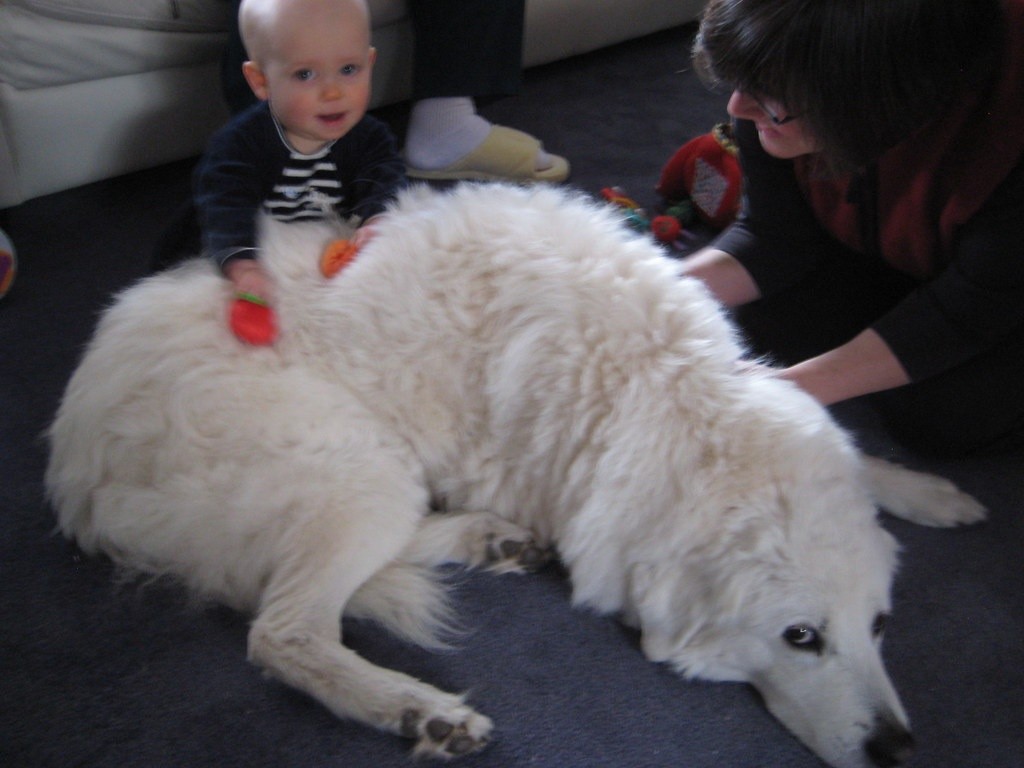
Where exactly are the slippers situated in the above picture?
[396,124,568,184]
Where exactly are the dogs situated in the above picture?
[47,180,988,767]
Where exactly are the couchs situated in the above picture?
[0,0,705,206]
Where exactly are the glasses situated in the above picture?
[746,90,811,126]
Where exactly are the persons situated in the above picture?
[675,0,1024,459]
[196,0,572,304]
